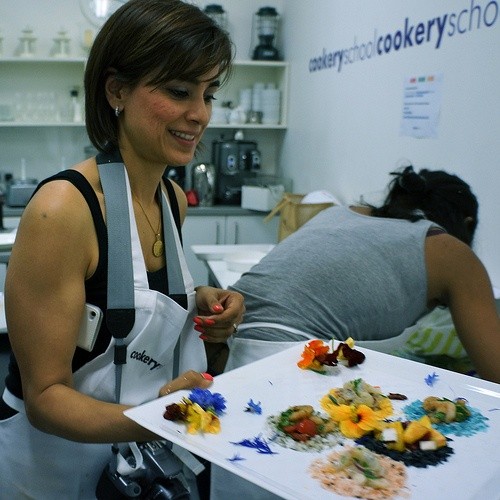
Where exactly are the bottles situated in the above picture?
[259,82,281,125]
[63,86,83,123]
[15,23,37,58]
[50,28,73,59]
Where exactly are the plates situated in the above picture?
[122,339,500,498]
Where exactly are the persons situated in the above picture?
[0,0,247,500]
[197,165,500,500]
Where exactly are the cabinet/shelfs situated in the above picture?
[180,214,280,290]
[184,61,287,217]
[1,58,98,231]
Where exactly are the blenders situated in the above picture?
[251,6,281,61]
[203,4,226,32]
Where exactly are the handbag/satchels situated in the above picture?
[264,193,334,242]
[95,440,205,500]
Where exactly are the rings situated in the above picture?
[233,323,239,333]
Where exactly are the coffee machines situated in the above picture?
[212,130,262,206]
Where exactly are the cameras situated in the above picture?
[95,440,191,500]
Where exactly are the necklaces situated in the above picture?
[131,184,163,257]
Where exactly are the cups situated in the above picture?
[15,90,34,121]
[37,91,60,122]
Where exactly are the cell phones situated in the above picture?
[77,303,104,352]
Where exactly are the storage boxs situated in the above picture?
[241,186,284,213]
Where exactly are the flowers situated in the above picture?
[298,337,366,376]
[164,387,227,436]
[266,377,471,500]
